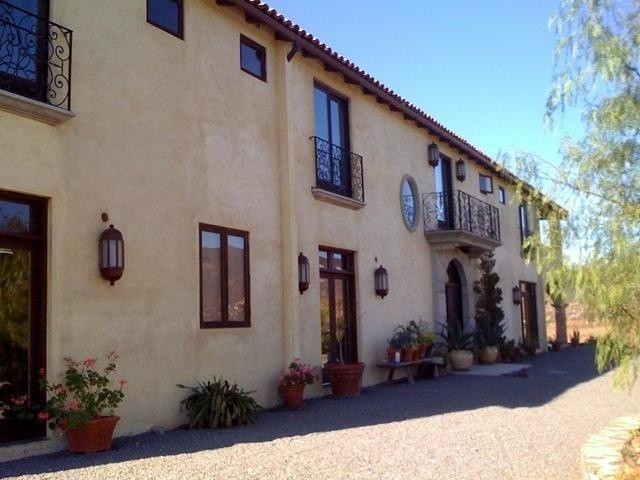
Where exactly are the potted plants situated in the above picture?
[469,317,512,365]
[321,312,365,396]
[385,318,435,361]
[434,317,481,373]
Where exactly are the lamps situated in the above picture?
[457,158,465,181]
[511,280,521,306]
[298,250,312,295]
[373,257,389,301]
[100,224,125,286]
[428,142,440,169]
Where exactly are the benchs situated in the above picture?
[374,356,444,384]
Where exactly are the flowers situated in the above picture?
[6,345,127,438]
[274,354,322,396]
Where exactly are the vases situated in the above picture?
[51,413,118,452]
[279,382,306,409]
